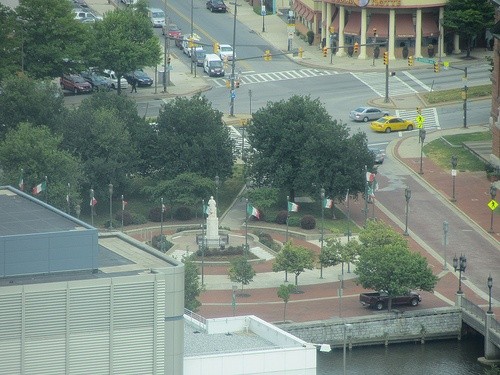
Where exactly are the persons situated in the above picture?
[130,78,138,94]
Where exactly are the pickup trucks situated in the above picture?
[360,287,422,311]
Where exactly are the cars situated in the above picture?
[206,0,226,13]
[85,67,153,88]
[72,0,104,25]
[59,74,91,95]
[162,24,226,78]
[369,115,415,133]
[349,107,390,122]
[219,44,236,62]
[370,150,384,164]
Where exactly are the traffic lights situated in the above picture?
[236,81,240,88]
[323,47,328,57]
[416,107,421,115]
[407,56,413,66]
[354,43,358,53]
[373,28,376,36]
[383,52,389,65]
[433,62,439,73]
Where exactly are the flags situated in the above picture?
[161,201,166,212]
[366,171,379,201]
[19,175,24,191]
[89,193,97,207]
[203,205,210,215]
[288,202,302,213]
[33,180,47,195]
[121,197,128,206]
[323,198,334,209]
[247,203,260,219]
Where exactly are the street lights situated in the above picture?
[402,187,412,236]
[449,154,458,201]
[485,274,496,328]
[452,252,467,308]
[489,183,497,233]
[418,127,426,176]
[442,221,449,270]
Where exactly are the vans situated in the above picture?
[148,8,166,28]
